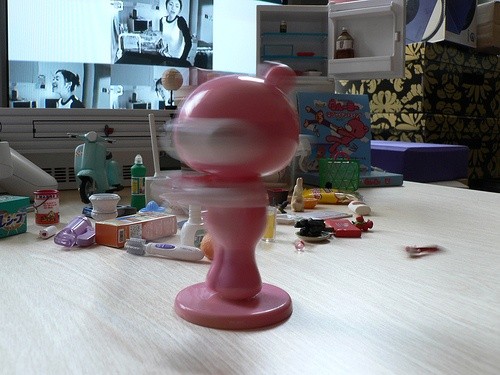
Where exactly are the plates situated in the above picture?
[303,72,322,76]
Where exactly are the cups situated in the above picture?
[280,20,287,32]
[147,178,170,202]
[262,205,276,242]
[34,189,61,225]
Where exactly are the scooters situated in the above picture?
[66,129,124,204]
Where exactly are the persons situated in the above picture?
[155,77,176,108]
[51,69,86,109]
[159,0,193,67]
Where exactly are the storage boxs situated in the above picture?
[335,0,500,183]
[0,194,30,238]
[94,211,177,248]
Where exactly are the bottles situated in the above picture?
[335,26,354,59]
[132,154,146,209]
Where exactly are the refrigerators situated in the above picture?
[255,0,406,108]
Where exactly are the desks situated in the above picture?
[0,179,500,375]
